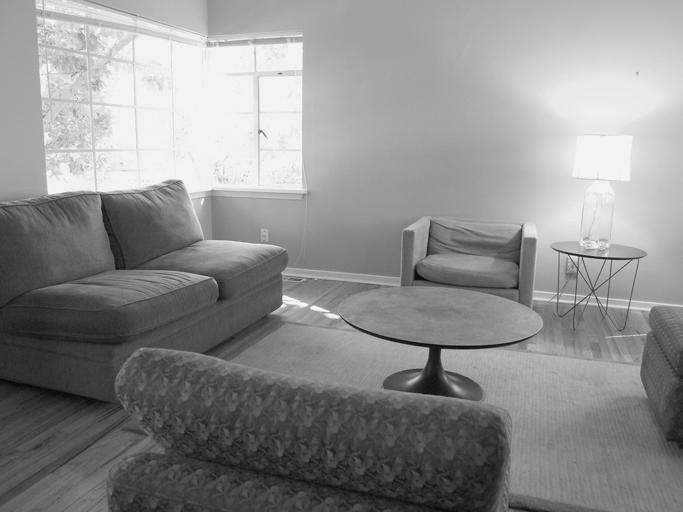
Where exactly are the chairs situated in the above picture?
[401,215,537,310]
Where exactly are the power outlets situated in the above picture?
[565,256,578,276]
[260,228,269,242]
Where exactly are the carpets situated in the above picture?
[216,320,683,512]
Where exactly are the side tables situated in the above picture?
[549,241,647,331]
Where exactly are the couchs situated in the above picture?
[106,347,511,512]
[639,304,683,448]
[0,179,289,404]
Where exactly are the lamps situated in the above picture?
[571,134,633,251]
